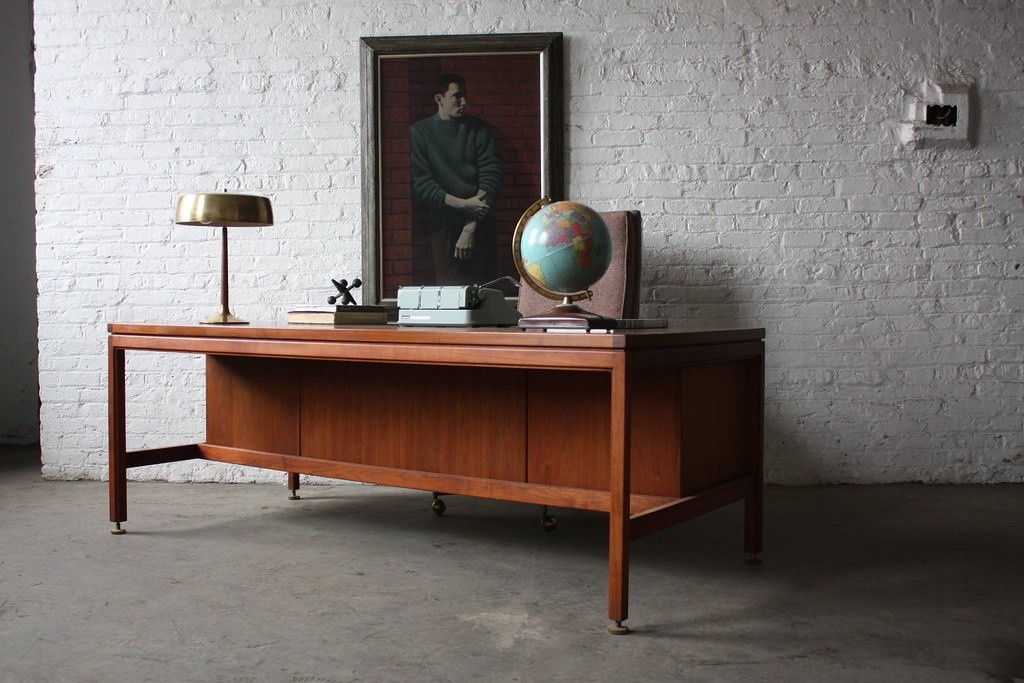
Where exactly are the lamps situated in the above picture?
[175,188,273,323]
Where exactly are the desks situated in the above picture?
[108,324,764,636]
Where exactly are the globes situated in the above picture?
[511,195,618,333]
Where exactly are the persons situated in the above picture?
[409,73,505,296]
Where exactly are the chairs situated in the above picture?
[432,210,641,530]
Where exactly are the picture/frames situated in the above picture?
[358,32,564,321]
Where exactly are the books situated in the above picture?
[614,319,669,330]
[287,304,388,326]
